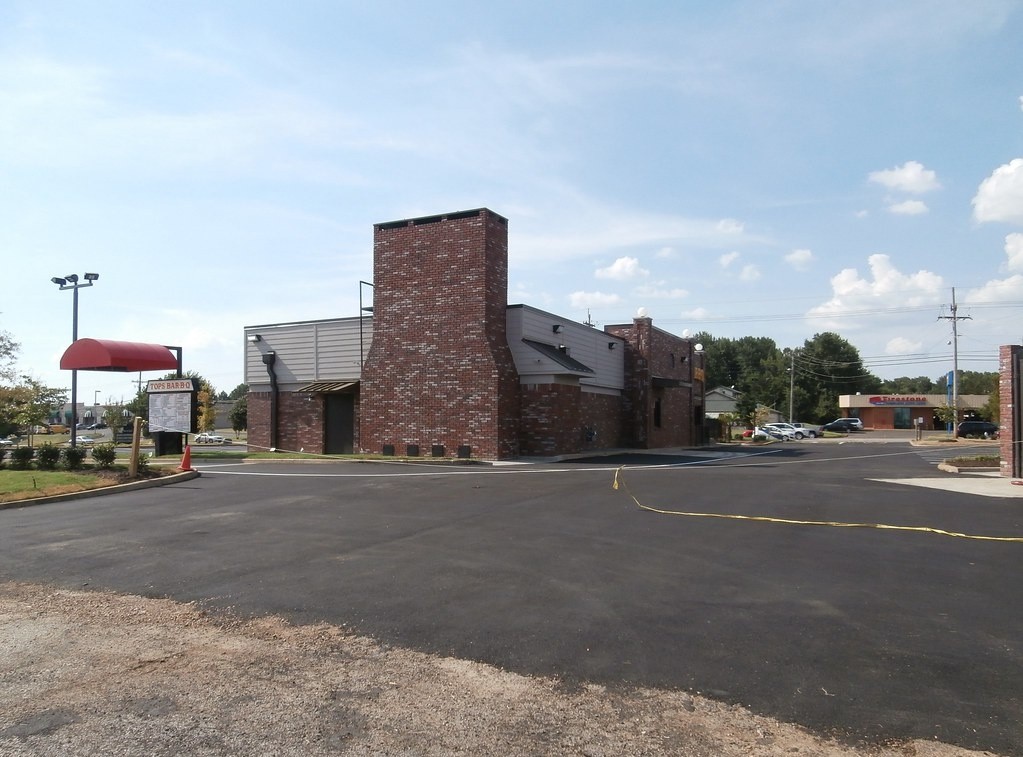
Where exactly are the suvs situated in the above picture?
[766,423,809,440]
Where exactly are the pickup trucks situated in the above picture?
[790,423,824,439]
[42,425,70,435]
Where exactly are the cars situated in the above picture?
[0,438,13,447]
[827,418,864,429]
[823,420,857,433]
[752,426,790,441]
[76,423,86,430]
[87,423,106,430]
[743,429,753,437]
[69,435,94,445]
[194,433,223,444]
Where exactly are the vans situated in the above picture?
[957,421,1000,439]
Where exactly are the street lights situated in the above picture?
[50,272,99,447]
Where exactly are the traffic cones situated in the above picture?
[177,445,194,471]
